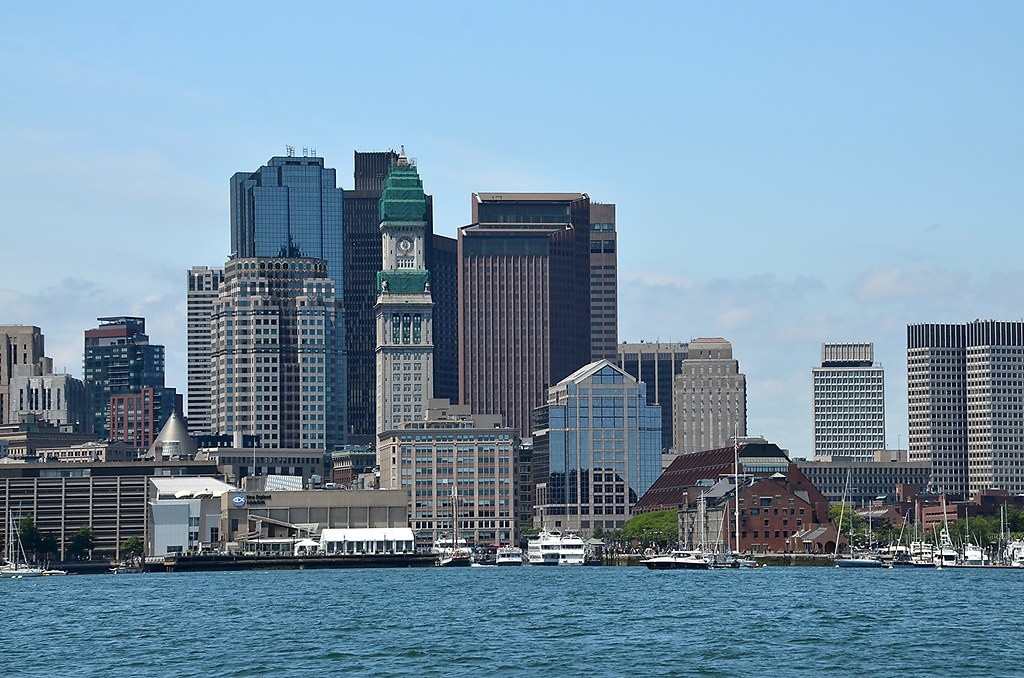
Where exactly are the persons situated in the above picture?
[897,551,910,561]
[711,547,772,555]
[622,547,671,554]
[808,548,825,555]
[961,556,970,566]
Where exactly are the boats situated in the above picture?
[496,543,524,566]
[108,557,148,576]
[526,521,587,567]
[41,570,68,577]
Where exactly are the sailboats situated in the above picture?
[0,504,44,577]
[640,489,760,570]
[437,485,475,568]
[833,465,1024,570]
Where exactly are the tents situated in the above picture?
[294,539,321,556]
